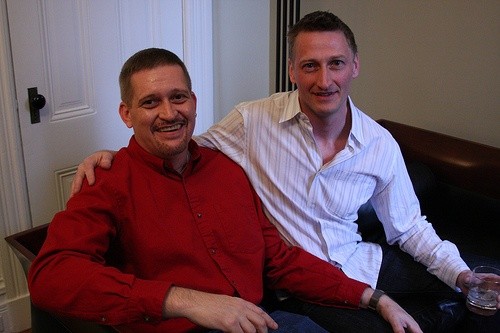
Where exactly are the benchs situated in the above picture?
[3,119,500,333]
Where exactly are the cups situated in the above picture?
[466,265,500,315]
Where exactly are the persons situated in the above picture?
[27,48,423,333]
[70,10,500,333]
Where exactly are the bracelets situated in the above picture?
[368,289,384,314]
